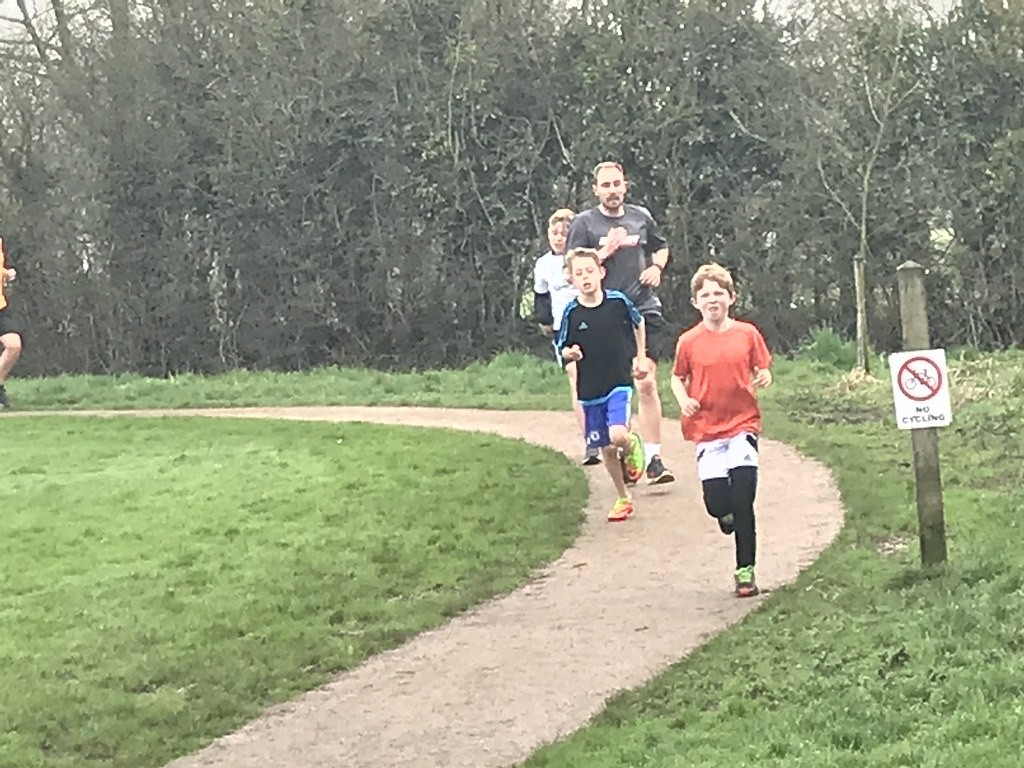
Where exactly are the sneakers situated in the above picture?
[582,443,602,465]
[607,494,634,521]
[733,565,758,597]
[623,433,644,480]
[718,513,735,535]
[0,385,10,410]
[615,446,639,487]
[645,454,675,485]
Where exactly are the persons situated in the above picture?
[561,162,675,486]
[557,248,648,522]
[533,209,602,465]
[0,238,22,407]
[671,264,772,596]
